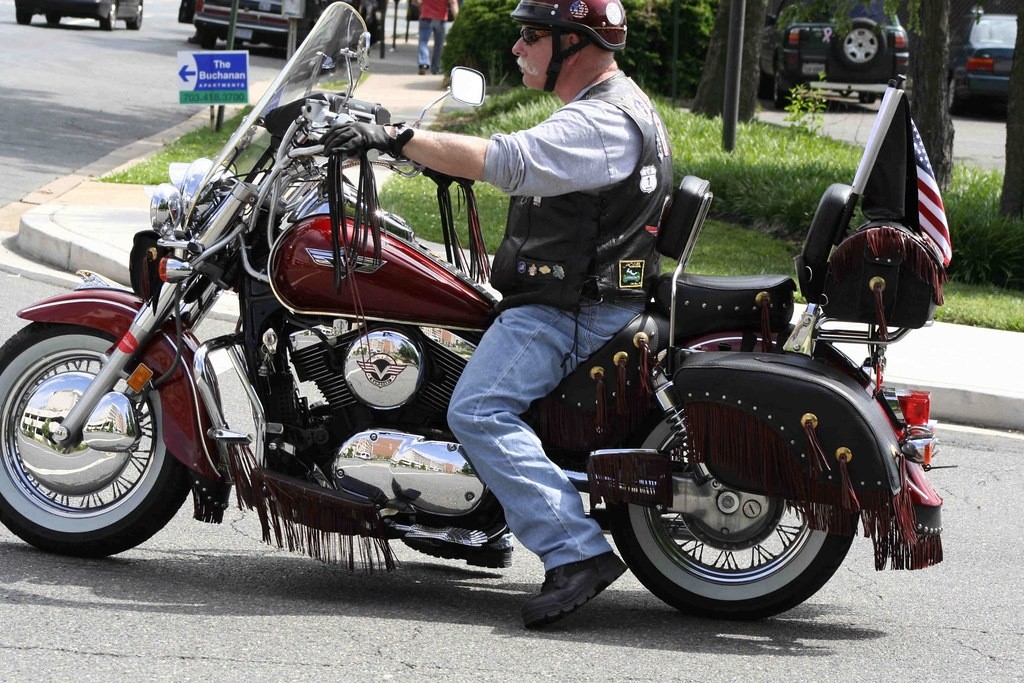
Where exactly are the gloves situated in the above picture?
[320,116,412,160]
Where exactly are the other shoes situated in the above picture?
[418,65,438,74]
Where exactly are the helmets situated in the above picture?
[511,1,629,49]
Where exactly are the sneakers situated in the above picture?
[524,550,630,627]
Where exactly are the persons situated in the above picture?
[318,0,674,631]
[418,0,459,75]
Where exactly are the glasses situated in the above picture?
[520,26,573,46]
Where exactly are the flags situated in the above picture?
[861,90,954,267]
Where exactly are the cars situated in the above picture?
[406,0,454,22]
[947,13,1019,118]
[15,0,144,31]
[192,0,387,52]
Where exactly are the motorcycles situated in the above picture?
[1,1,953,622]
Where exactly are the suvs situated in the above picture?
[759,0,912,112]
